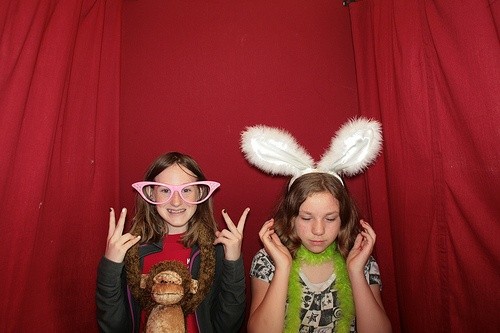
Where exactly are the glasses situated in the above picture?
[130,178,220,207]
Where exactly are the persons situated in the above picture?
[248,168,393,333]
[95,152,251,332]
